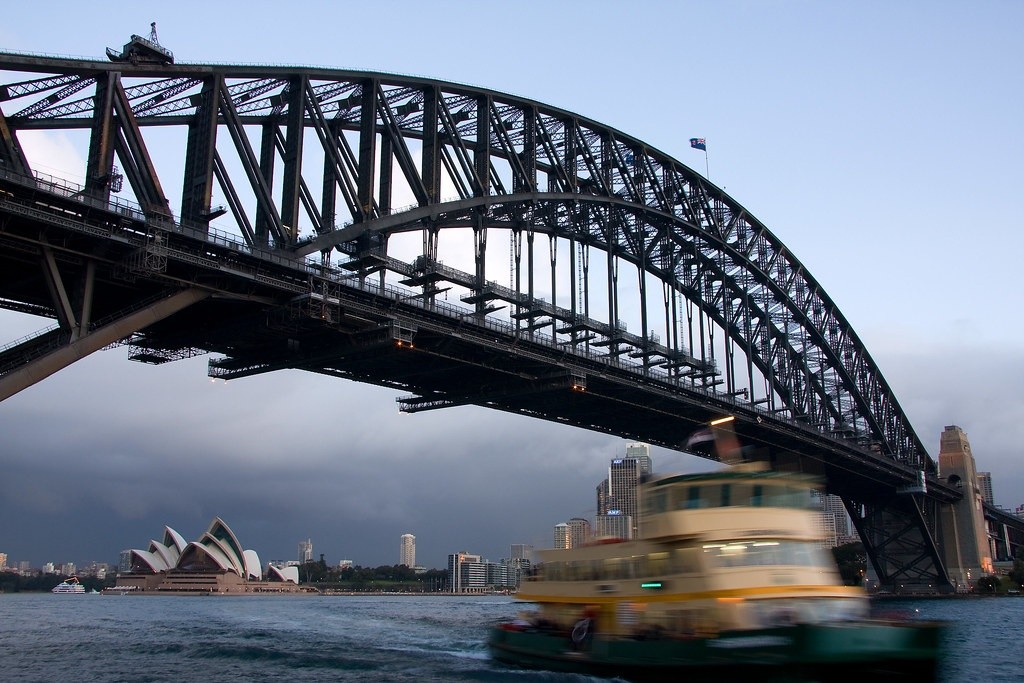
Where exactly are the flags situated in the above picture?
[690,138,706,150]
[627,155,635,164]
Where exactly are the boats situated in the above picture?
[52,576,85,594]
[478,418,963,683]
